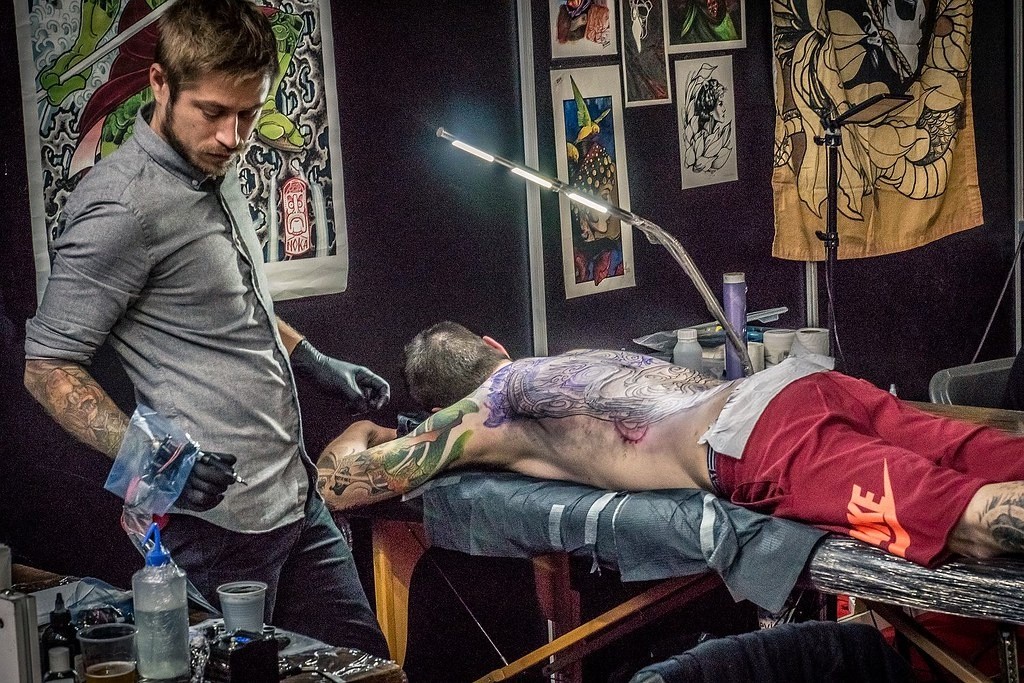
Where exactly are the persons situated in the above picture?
[24,2,392,662]
[315,319,1024,572]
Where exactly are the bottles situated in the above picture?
[43,647,82,683]
[672,327,703,374]
[722,272,749,381]
[41,591,85,683]
[132,523,194,683]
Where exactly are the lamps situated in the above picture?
[434,127,758,377]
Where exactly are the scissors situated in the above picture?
[296,656,347,683]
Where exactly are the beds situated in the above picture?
[347,465,1024,683]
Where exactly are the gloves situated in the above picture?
[290,341,392,416]
[136,441,237,511]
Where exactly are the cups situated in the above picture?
[77,622,140,683]
[215,580,267,635]
